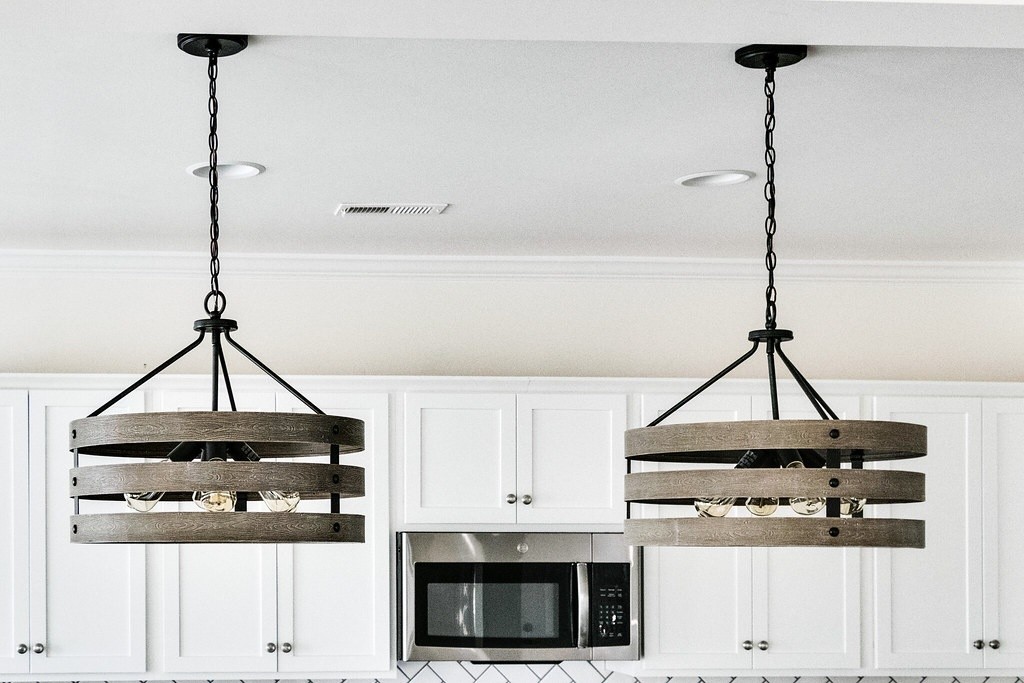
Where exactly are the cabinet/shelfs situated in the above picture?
[397,375,634,532]
[1,372,156,682]
[156,374,397,679]
[867,380,1024,677]
[632,377,867,676]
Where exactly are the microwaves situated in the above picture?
[397,533,641,662]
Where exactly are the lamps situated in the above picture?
[69,30,928,548]
